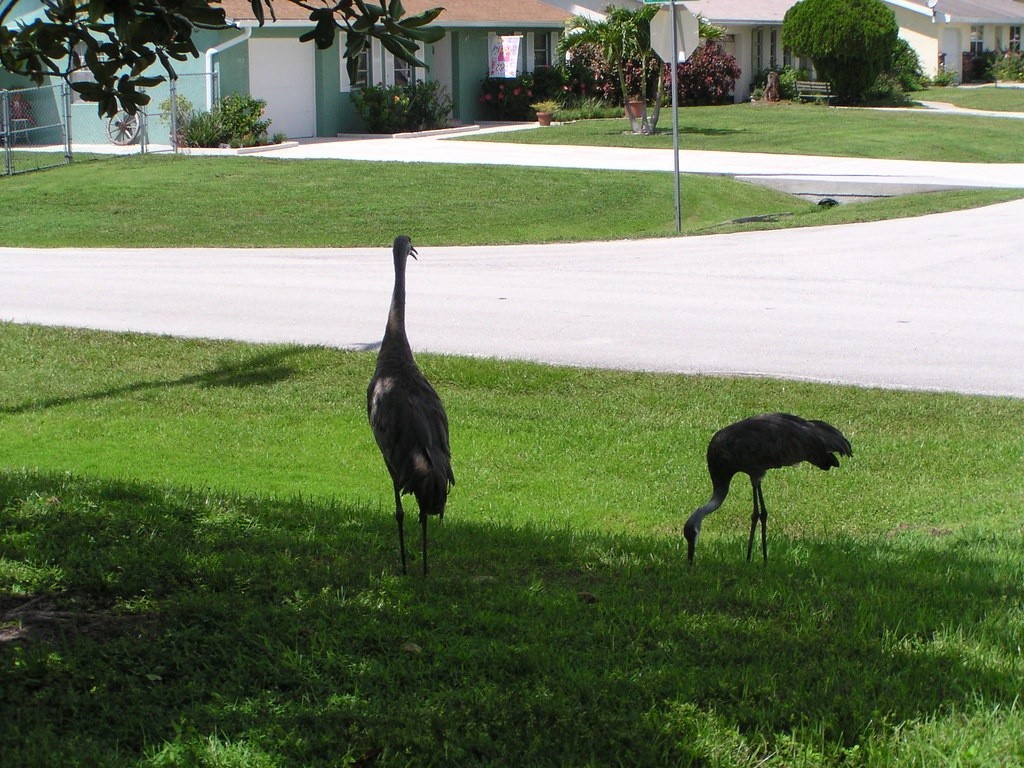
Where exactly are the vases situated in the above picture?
[623,99,643,118]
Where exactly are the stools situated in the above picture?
[10,118,31,147]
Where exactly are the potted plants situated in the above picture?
[528,98,563,127]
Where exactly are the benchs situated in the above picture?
[795,79,839,106]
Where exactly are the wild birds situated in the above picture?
[366,235,456,575]
[684,412,853,567]
